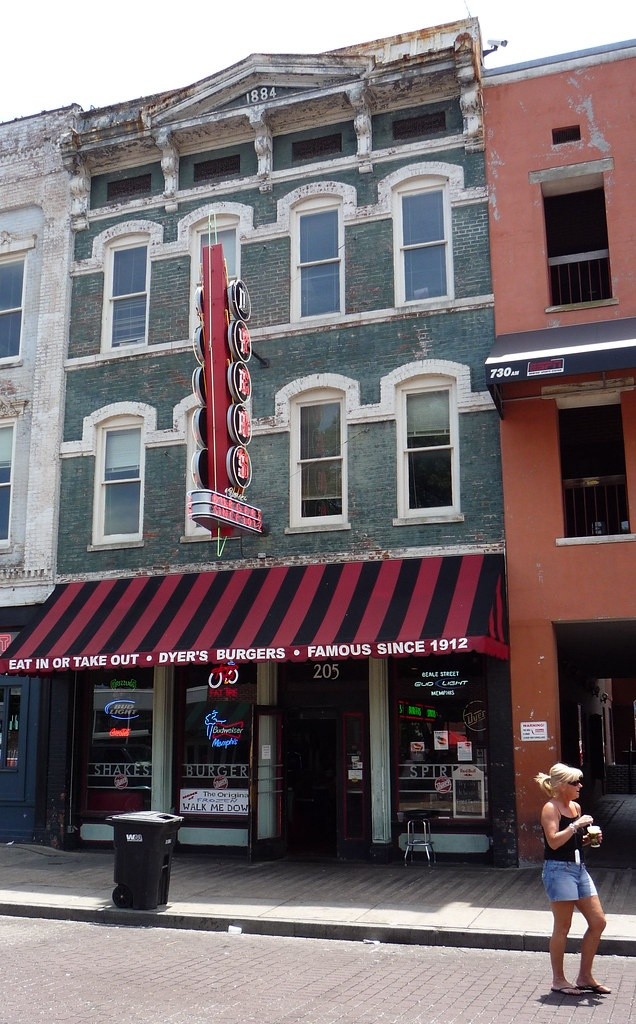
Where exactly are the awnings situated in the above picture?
[0,554,510,675]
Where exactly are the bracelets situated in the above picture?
[569,823,578,833]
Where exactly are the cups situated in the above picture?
[587,826,600,848]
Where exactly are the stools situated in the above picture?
[404,810,437,867]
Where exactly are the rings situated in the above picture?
[589,816,591,818]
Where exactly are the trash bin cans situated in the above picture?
[105,811,184,910]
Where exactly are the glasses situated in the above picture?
[567,780,581,786]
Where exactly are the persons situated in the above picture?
[534,763,612,995]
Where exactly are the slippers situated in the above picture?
[551,986,585,995]
[576,984,611,995]
